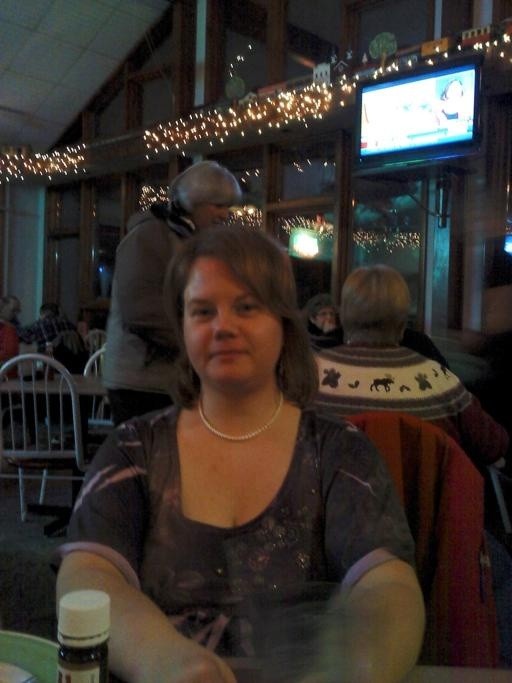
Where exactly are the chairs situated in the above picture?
[0,329,115,534]
[344,412,501,668]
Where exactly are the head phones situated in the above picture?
[147,201,195,238]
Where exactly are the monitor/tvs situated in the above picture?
[353,54,480,169]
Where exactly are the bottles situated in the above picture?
[57,590,110,683]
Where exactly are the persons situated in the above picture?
[50,218,431,682]
[101,159,246,431]
[2,294,104,446]
[440,78,463,120]
[303,263,509,464]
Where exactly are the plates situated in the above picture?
[0,631,59,683]
